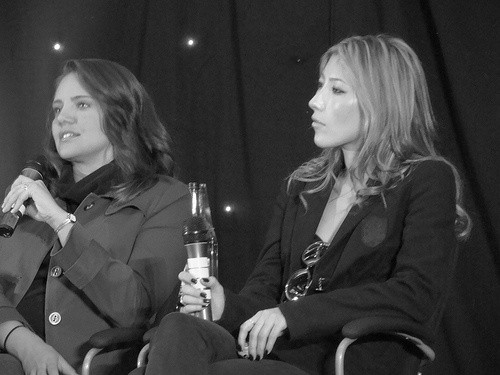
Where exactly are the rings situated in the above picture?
[23,182,29,189]
[248,317,256,325]
[180,294,185,306]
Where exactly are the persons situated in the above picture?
[0,57,199,375]
[141,34,471,375]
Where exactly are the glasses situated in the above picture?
[285,241,332,303]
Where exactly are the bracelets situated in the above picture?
[4,324,26,353]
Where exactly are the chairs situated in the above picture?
[127,204,473,375]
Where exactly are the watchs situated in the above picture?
[55,212,76,234]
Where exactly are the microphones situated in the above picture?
[183,216,213,323]
[0,159,47,236]
